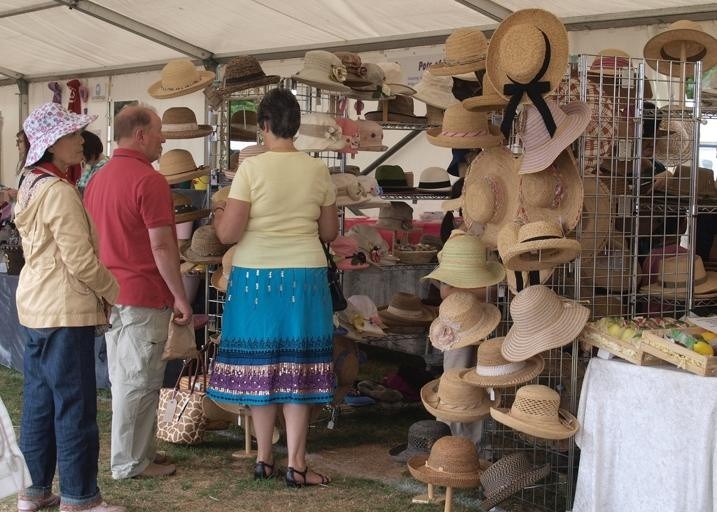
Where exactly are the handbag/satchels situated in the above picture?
[94,297,112,339]
[156,351,210,445]
[319,235,348,312]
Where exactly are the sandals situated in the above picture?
[253,460,279,482]
[286,466,333,488]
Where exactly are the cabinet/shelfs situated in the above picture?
[202,77,479,419]
[479,54,716,511]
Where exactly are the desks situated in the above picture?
[0,272,111,391]
[571,355,716,511]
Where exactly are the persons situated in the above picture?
[204,88,340,487]
[77,131,108,188]
[626,102,687,296]
[439,145,496,459]
[14,103,127,511]
[14,130,30,189]
[82,105,193,481]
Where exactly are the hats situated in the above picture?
[151,148,212,183]
[642,18,716,78]
[407,434,496,488]
[230,110,259,142]
[178,226,233,264]
[218,54,281,93]
[223,145,270,179]
[168,191,212,224]
[294,10,592,439]
[595,79,716,301]
[589,47,635,76]
[20,101,99,169]
[145,59,217,100]
[476,451,552,512]
[211,186,232,203]
[389,419,453,464]
[0,188,18,223]
[156,107,213,140]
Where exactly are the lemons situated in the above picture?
[702,332,716,343]
[693,341,714,355]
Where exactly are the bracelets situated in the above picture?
[213,208,223,213]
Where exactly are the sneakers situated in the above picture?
[153,451,168,463]
[112,461,177,478]
[59,498,129,511]
[17,493,60,511]
